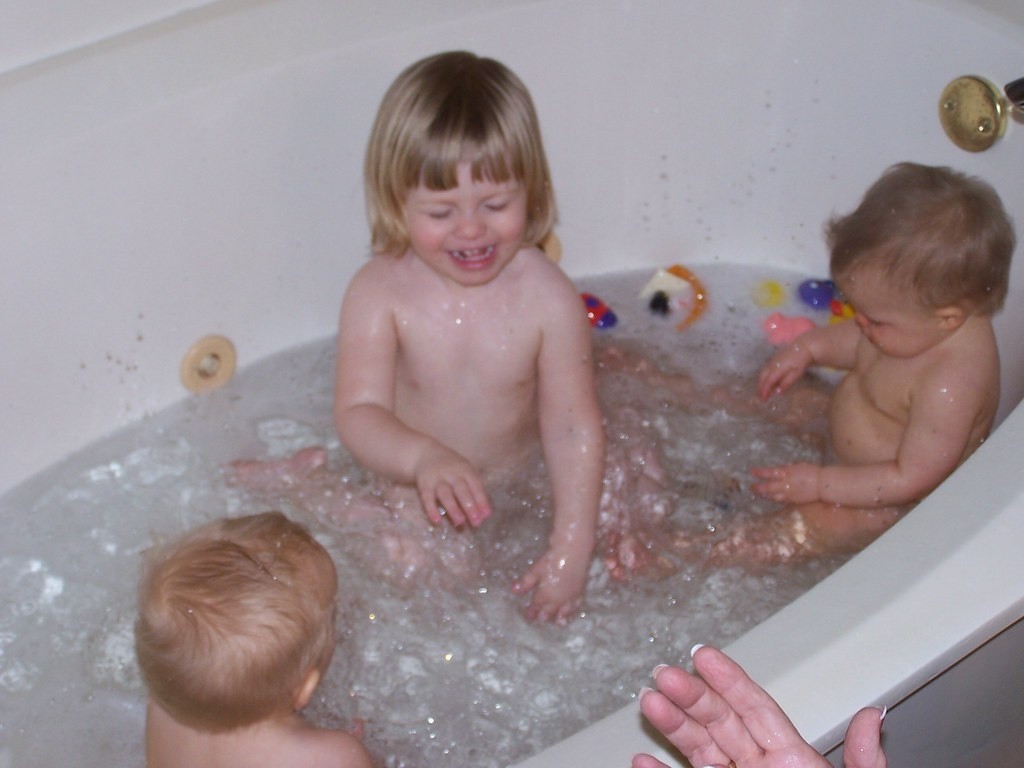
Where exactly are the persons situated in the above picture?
[133,511,377,768]
[220,52,604,627]
[628,642,893,767]
[607,163,1015,580]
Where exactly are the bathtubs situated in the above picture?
[2,0,1023,767]
